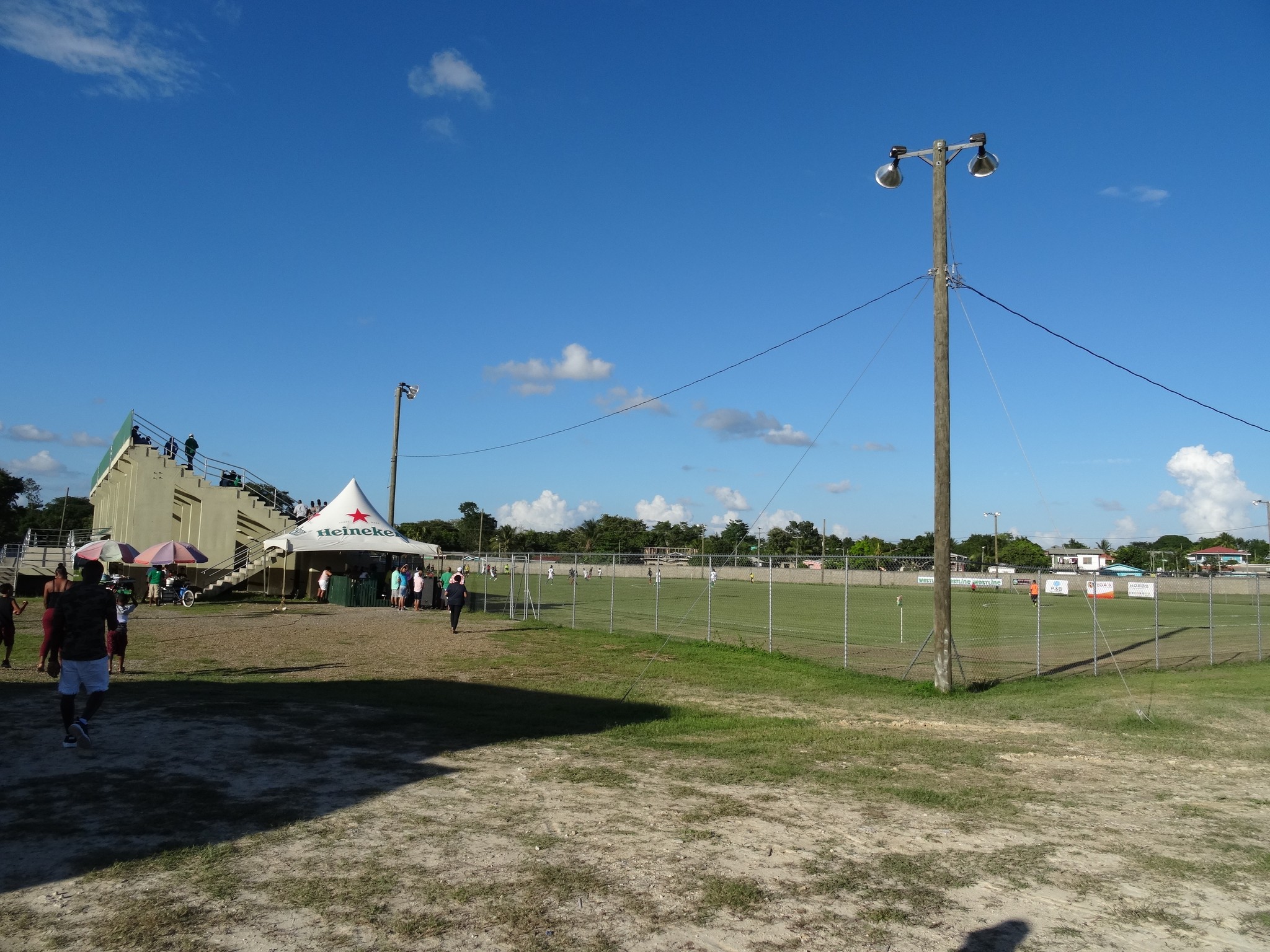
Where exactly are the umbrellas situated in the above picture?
[71,539,209,576]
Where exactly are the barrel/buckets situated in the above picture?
[166,578,174,587]
[179,588,188,598]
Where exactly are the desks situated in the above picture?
[100,580,136,584]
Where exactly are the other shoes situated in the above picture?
[37,663,44,672]
[108,669,112,674]
[1,660,12,668]
[118,668,125,673]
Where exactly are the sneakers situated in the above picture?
[63,735,77,748]
[68,717,92,749]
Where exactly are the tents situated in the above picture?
[263,478,439,602]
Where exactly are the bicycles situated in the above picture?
[173,584,195,607]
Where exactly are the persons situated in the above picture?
[750,573,754,583]
[133,425,200,471]
[219,470,247,491]
[293,499,328,527]
[545,566,717,588]
[1029,580,1039,608]
[316,563,509,635]
[0,560,189,749]
[971,582,975,592]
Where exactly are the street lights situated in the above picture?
[983,512,1001,590]
[387,382,420,528]
[836,548,844,556]
[874,130,999,698]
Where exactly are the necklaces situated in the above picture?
[56,577,64,578]
